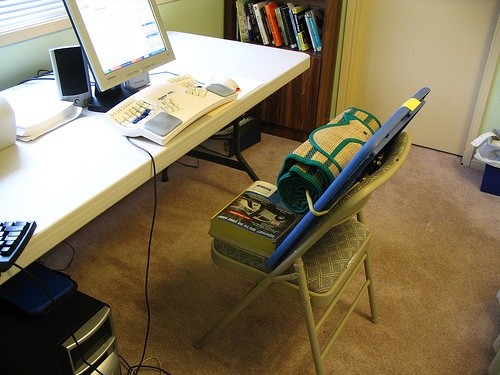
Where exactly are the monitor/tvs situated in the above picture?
[62,0,176,114]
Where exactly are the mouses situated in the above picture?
[210,76,240,92]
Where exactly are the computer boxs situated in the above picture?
[1,289,123,375]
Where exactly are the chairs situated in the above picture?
[193,87,431,375]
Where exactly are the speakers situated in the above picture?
[49,44,92,108]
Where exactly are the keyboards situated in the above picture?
[104,73,239,146]
[0,220,36,273]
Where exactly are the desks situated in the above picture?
[0,30,310,287]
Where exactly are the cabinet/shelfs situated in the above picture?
[224,0,342,143]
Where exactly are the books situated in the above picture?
[236,0,322,52]
[208,180,303,259]
